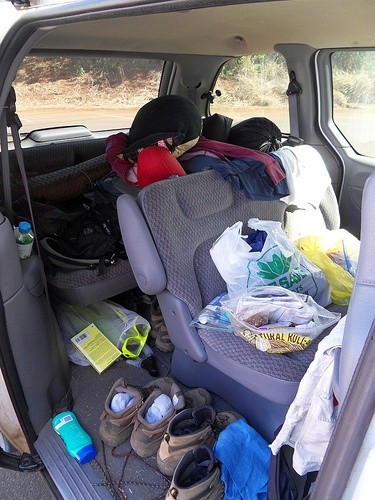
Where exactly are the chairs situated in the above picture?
[22,144,362,439]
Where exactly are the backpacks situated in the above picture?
[16,199,128,272]
[80,171,138,234]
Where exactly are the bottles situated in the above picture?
[13,221,33,263]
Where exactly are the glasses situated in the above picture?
[121,317,150,358]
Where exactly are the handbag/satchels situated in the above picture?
[209,218,333,308]
[294,229,361,305]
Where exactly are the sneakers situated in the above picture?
[131,387,211,457]
[98,378,176,446]
[149,297,163,338]
[157,406,237,477]
[154,316,174,352]
[165,446,225,500]
